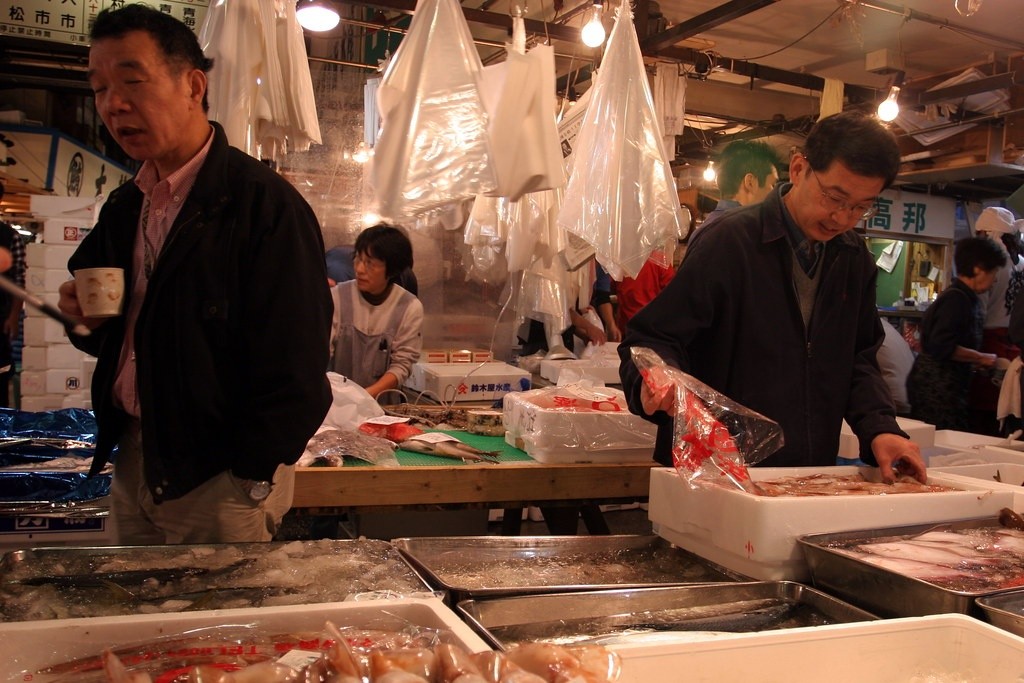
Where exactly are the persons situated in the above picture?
[0,181,26,408]
[58,3,334,545]
[617,109,927,484]
[877,207,1024,417]
[905,236,1007,432]
[523,140,781,356]
[326,224,424,405]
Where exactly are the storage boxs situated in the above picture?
[0,220,1024,683]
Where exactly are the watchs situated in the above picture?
[235,476,272,501]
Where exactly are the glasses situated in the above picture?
[814,168,880,221]
[354,254,386,271]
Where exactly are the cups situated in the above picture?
[73,267,124,317]
